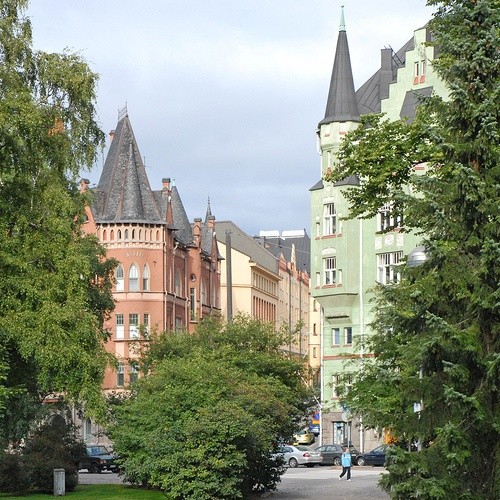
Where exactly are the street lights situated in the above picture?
[272,350,323,452]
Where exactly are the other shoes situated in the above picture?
[339,477,342,480]
[347,479,351,481]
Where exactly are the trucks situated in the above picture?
[309,411,320,436]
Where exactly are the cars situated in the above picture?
[262,445,323,468]
[354,445,414,466]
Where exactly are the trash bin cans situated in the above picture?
[53,468,66,496]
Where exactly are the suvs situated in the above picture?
[80,445,121,474]
[293,427,315,445]
[313,444,360,467]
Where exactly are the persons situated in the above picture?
[339,448,353,481]
